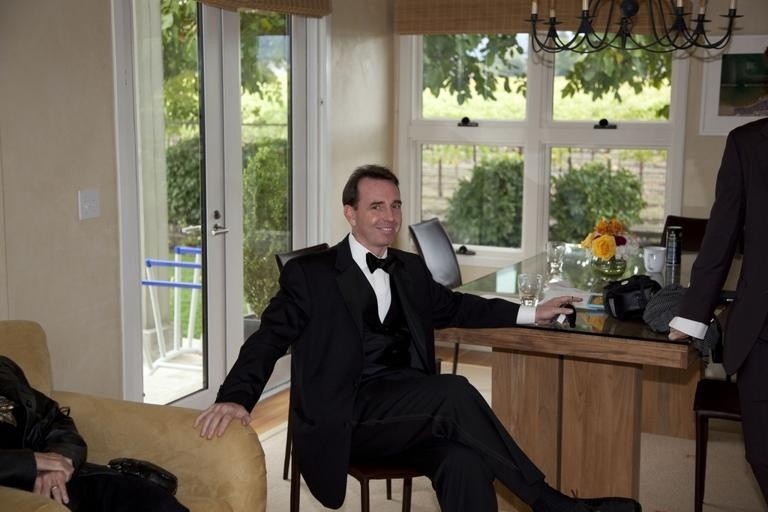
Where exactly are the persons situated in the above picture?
[0,354,191,512]
[666,119,768,504]
[191,163,643,512]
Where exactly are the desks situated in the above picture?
[433,241,744,512]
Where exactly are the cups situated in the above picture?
[643,247,666,274]
[518,273,541,307]
[547,241,566,274]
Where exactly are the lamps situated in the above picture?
[523,0,746,54]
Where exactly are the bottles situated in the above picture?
[665,226,683,267]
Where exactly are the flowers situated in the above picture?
[580,216,639,260]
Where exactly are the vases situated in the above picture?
[590,255,627,276]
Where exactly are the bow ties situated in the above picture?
[365,252,392,273]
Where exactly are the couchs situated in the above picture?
[0,319,268,512]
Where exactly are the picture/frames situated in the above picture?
[698,34,768,137]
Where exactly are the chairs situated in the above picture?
[692,378,742,512]
[661,214,746,255]
[408,217,463,375]
[290,436,425,512]
[275,243,330,480]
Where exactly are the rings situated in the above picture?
[51,484,59,489]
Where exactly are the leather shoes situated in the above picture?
[569,489,642,512]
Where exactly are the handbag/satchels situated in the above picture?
[109,457,178,497]
